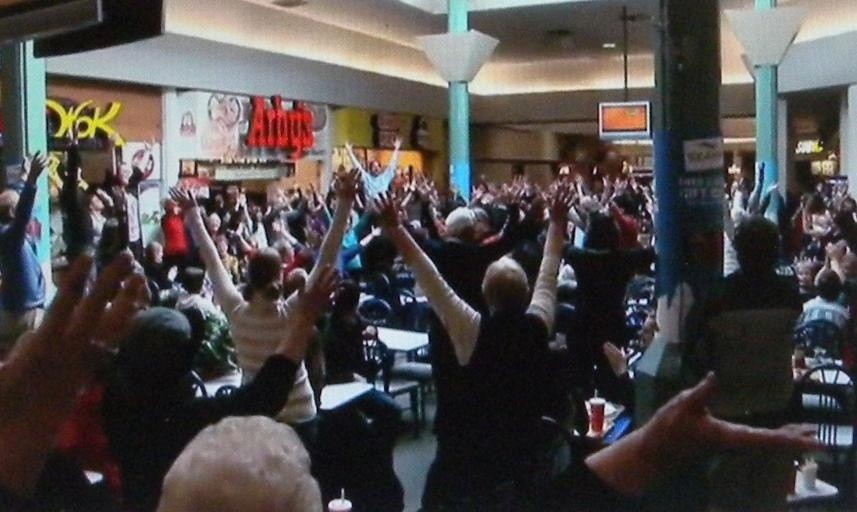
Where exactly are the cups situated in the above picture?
[803,461,818,490]
[327,499,352,512]
[590,398,606,431]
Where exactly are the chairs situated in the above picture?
[790,364,857,461]
[190,369,239,398]
[357,271,433,441]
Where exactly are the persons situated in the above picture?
[0,137,857,512]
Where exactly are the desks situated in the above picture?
[320,380,373,412]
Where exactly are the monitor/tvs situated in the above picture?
[597,101,652,139]
[1,0,169,58]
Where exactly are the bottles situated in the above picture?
[794,344,805,369]
